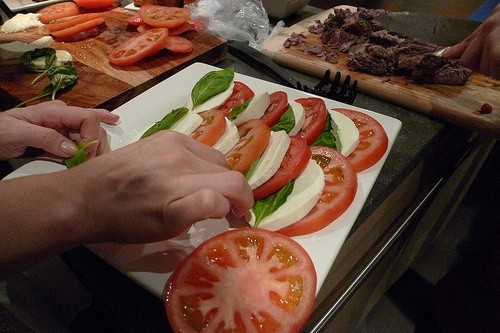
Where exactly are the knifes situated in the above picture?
[372,11,483,47]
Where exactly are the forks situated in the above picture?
[310,68,359,105]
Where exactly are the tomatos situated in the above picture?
[110,4,196,64]
[275,145,357,236]
[187,109,226,147]
[216,81,254,116]
[223,119,270,174]
[164,227,316,333]
[259,91,288,127]
[250,134,311,198]
[294,98,327,144]
[37,0,117,41]
[328,108,389,173]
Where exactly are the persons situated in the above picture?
[0,100,255,278]
[442,3,500,78]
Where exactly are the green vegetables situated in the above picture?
[142,67,342,227]
[14,46,78,107]
[62,138,98,168]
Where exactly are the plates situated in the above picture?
[1,63,403,297]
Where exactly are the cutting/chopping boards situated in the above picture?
[260,4,500,130]
[0,11,228,108]
